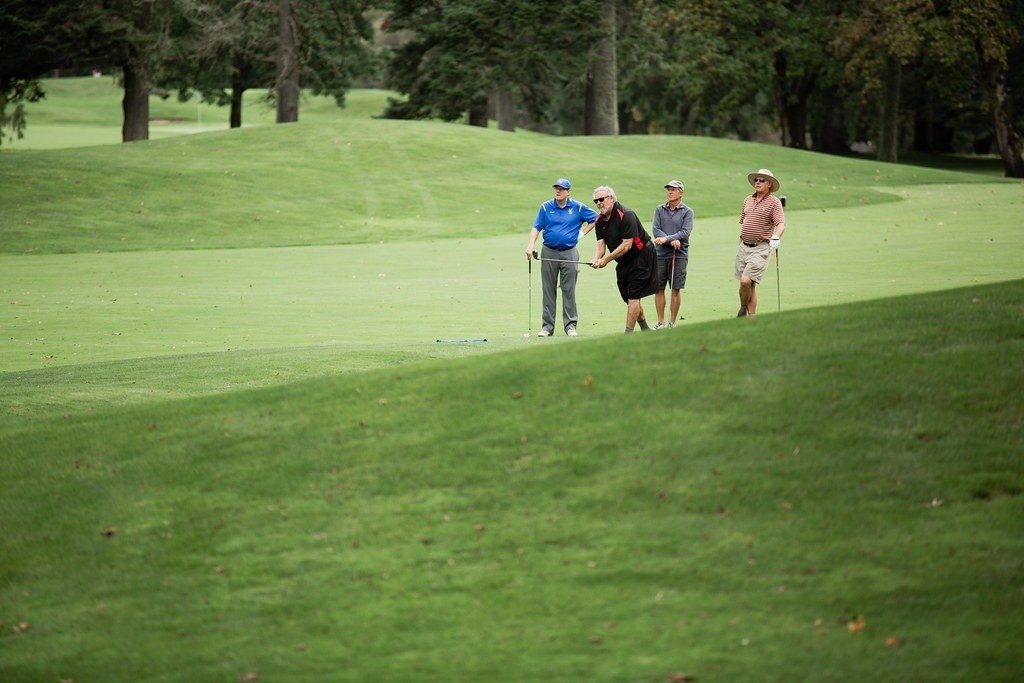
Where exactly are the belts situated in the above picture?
[543,243,575,251]
[743,242,757,247]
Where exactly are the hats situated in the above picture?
[664,180,684,191]
[748,169,779,192]
[552,179,571,190]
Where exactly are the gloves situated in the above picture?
[770,235,780,249]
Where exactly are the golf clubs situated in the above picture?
[523,260,531,338]
[666,249,677,328]
[532,249,592,267]
[775,247,781,313]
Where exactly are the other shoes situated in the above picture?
[652,322,666,330]
[667,322,676,329]
[566,328,577,336]
[538,331,551,336]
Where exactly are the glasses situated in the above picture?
[593,196,609,203]
[755,178,769,182]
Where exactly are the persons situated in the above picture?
[652,180,694,330]
[525,178,600,337]
[734,169,785,317]
[591,186,659,333]
